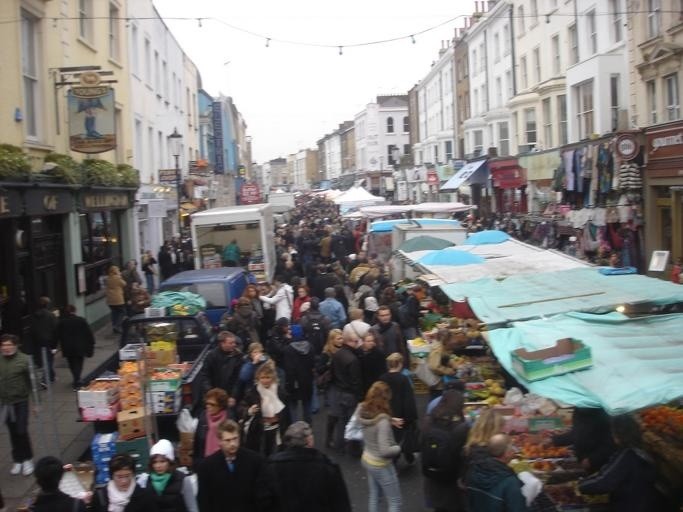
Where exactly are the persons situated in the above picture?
[343,381,404,510]
[28,296,59,389]
[529,226,622,268]
[104,266,127,332]
[199,419,270,511]
[1,335,35,475]
[137,439,197,510]
[197,191,525,465]
[158,232,194,278]
[29,456,87,510]
[265,422,352,511]
[462,433,530,510]
[56,305,95,391]
[123,250,156,315]
[91,454,156,510]
[542,405,671,511]
[222,239,241,267]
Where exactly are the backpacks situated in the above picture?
[421,430,454,478]
[308,319,323,335]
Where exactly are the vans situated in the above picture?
[158,266,255,331]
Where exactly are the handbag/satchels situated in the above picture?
[312,369,333,387]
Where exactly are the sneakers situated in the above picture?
[69,382,79,392]
[21,461,32,475]
[10,462,19,474]
[50,374,57,382]
[38,377,47,387]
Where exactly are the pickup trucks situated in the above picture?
[73,310,222,439]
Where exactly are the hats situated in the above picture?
[291,325,304,334]
[363,297,379,310]
[148,439,174,462]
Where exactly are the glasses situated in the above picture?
[205,403,218,408]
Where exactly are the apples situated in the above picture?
[80,341,178,392]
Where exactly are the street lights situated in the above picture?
[166,127,186,238]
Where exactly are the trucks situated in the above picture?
[266,192,297,231]
[190,201,278,287]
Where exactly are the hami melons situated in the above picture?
[485,379,504,409]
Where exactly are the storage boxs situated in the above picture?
[502,416,562,432]
[511,337,592,382]
[201,244,266,283]
[407,339,434,395]
[77,350,183,483]
